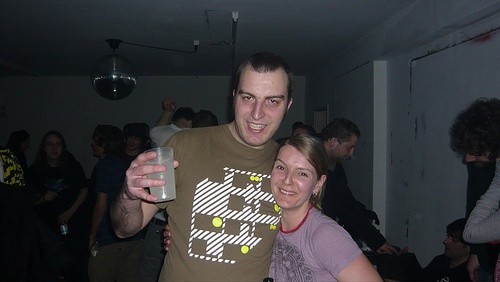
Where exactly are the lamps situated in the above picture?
[91,39,137,100]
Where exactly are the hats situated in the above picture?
[123,123,149,138]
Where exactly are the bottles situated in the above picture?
[59,220,68,235]
[89,241,98,261]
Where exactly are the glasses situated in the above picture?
[463,140,487,157]
[92,134,106,147]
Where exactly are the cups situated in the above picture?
[143,148,176,202]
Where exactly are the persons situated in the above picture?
[32,131,88,282]
[121,122,152,162]
[139,98,195,282]
[110,52,294,282]
[363,218,494,282]
[88,125,142,282]
[163,134,384,282]
[448,97,500,282]
[316,117,399,255]
